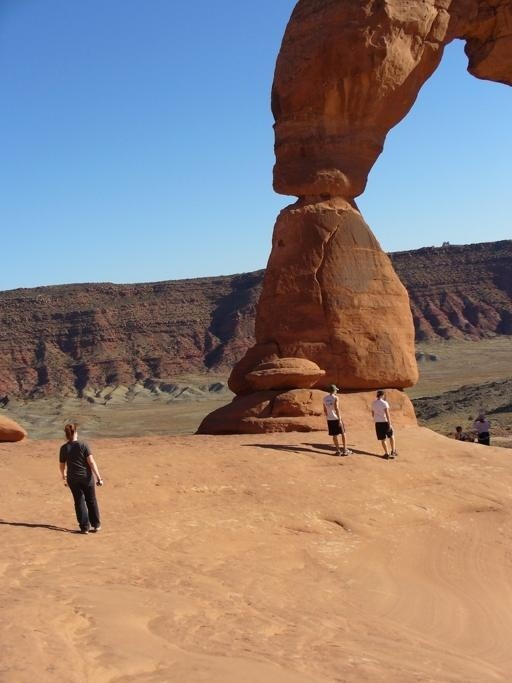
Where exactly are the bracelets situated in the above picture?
[98,478,102,481]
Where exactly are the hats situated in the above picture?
[330,384,339,391]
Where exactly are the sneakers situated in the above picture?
[383,454,388,459]
[335,452,340,456]
[94,527,101,531]
[80,530,88,534]
[391,451,398,456]
[343,450,352,455]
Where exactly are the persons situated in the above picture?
[455,427,469,440]
[323,384,352,456]
[371,391,397,458]
[474,415,491,446]
[59,423,103,536]
